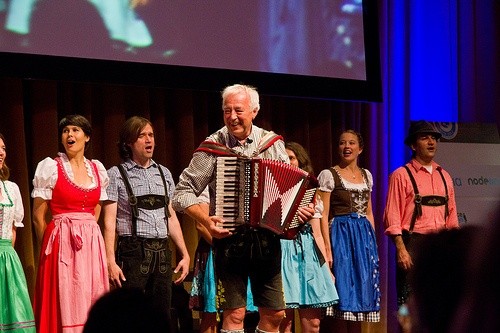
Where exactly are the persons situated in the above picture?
[0,135,37,333]
[280,141,340,333]
[104,117,190,286]
[317,127,381,333]
[383,121,460,333]
[81,285,170,332]
[31,114,107,333]
[172,85,315,333]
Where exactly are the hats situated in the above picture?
[405,120,441,148]
